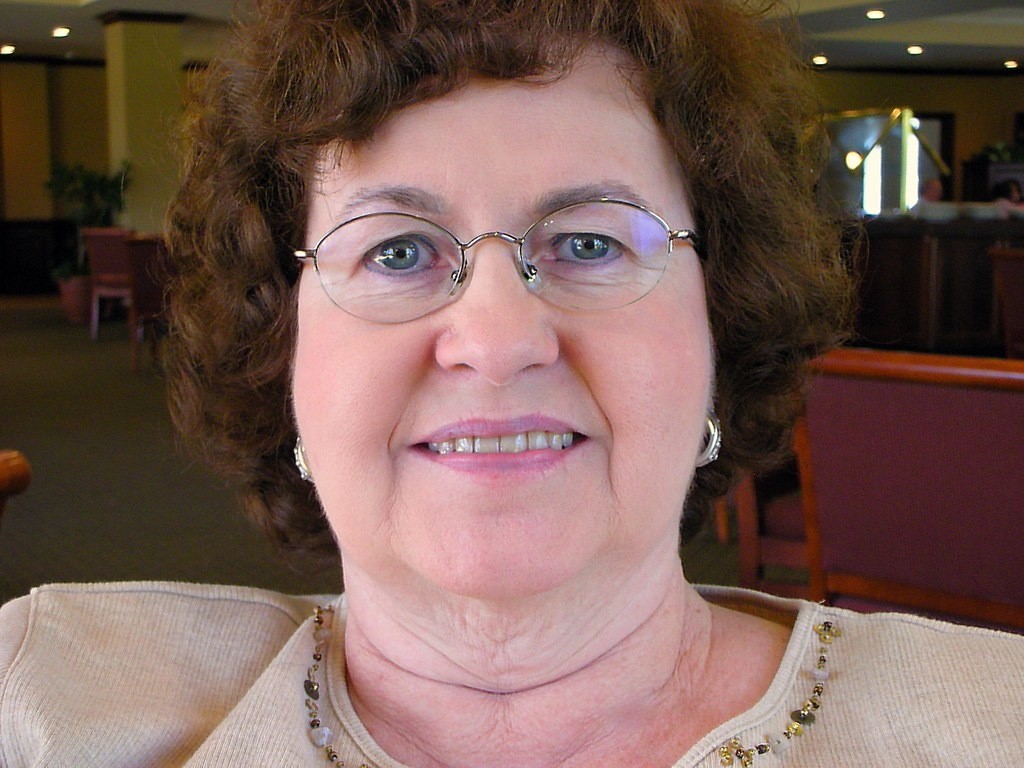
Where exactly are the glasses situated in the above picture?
[291,198,709,325]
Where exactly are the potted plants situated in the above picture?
[44,158,134,325]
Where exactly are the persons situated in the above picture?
[0,1,1024,768]
[908,170,1024,219]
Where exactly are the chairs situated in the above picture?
[989,247,1024,359]
[736,473,807,599]
[82,226,138,337]
[793,348,1024,635]
[125,234,177,373]
